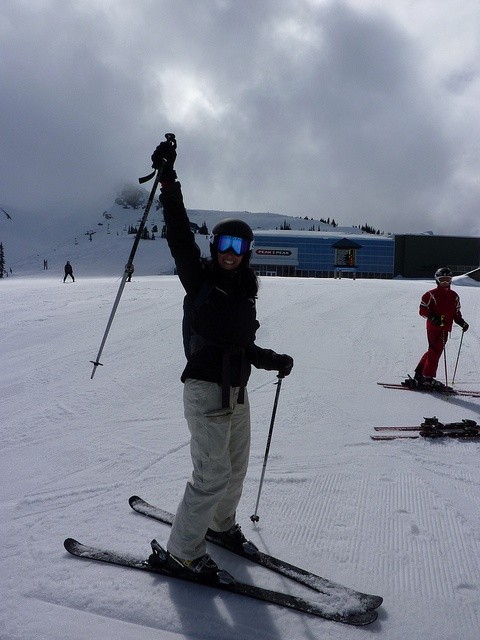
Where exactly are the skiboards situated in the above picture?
[64,496,383,627]
[377,382,479,397]
[370,417,479,441]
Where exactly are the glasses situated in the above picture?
[435,276,452,284]
[216,234,251,257]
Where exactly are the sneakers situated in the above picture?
[419,377,443,389]
[414,373,424,382]
[166,549,219,579]
[207,524,245,547]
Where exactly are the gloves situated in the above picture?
[461,322,469,332]
[151,142,177,182]
[429,315,445,326]
[265,349,293,376]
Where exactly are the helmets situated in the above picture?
[210,219,254,266]
[434,268,452,284]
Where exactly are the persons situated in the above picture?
[63,261,76,282]
[125,263,135,282]
[149,140,294,577]
[412,267,469,387]
